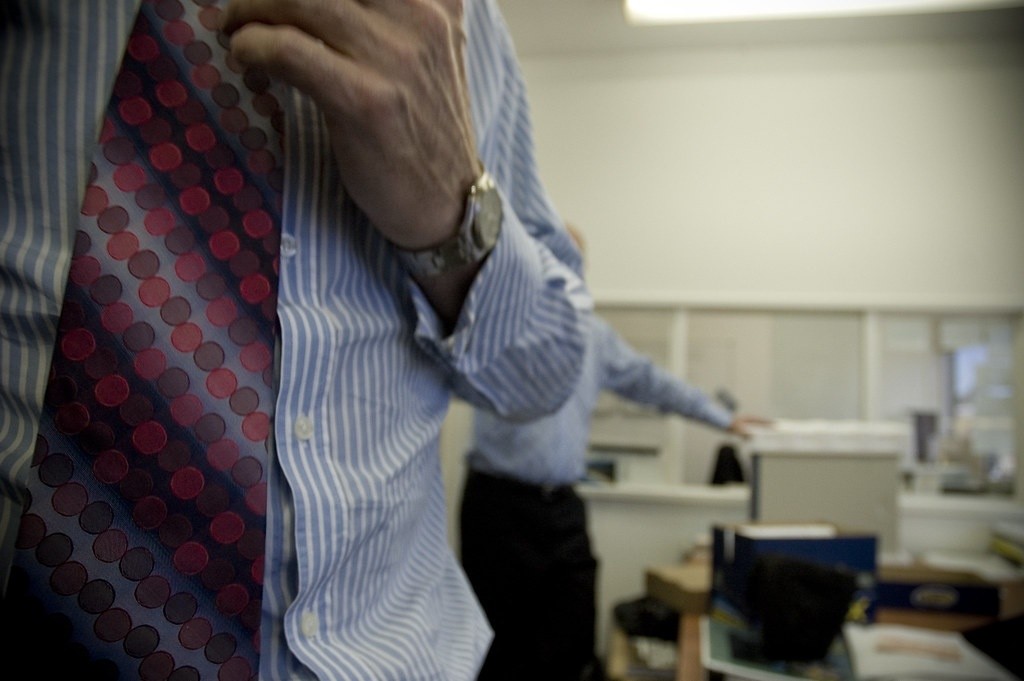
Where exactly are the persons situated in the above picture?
[0,0,588,681]
[458,224,752,681]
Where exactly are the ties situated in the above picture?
[0,0,292,681]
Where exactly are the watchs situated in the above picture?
[396,157,505,279]
